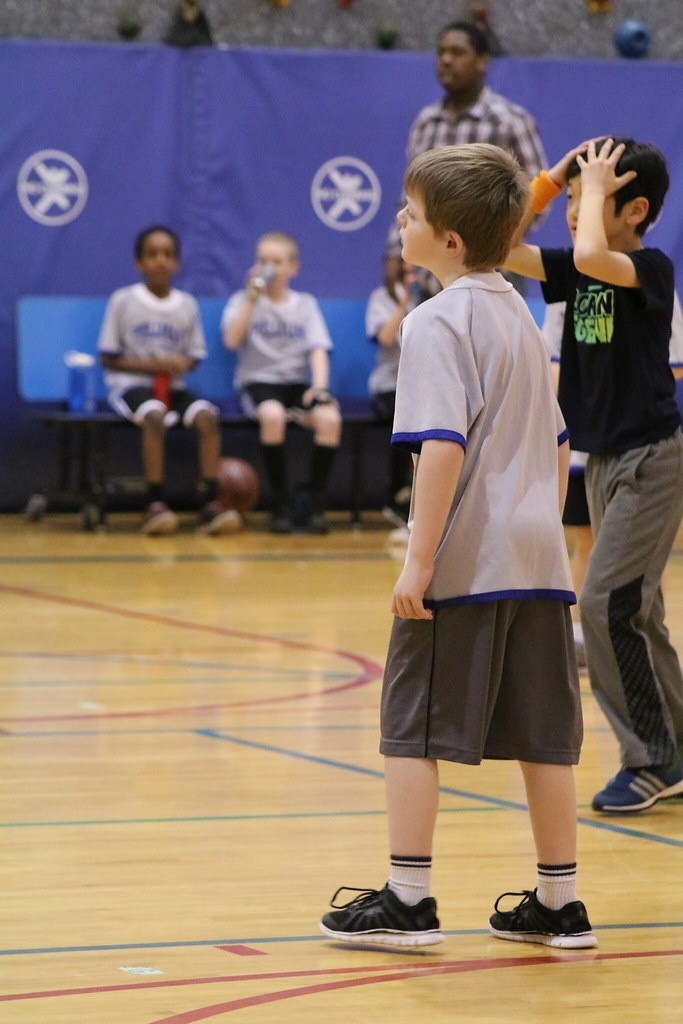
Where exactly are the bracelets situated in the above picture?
[527,170,561,214]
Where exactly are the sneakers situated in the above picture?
[487,886,599,949]
[319,880,445,948]
[591,750,683,813]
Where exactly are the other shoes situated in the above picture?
[294,488,329,535]
[382,501,409,528]
[197,499,245,537]
[141,500,181,535]
[270,485,294,533]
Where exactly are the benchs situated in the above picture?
[19,405,402,539]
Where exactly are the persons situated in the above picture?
[505,137,683,811]
[386,21,549,307]
[543,286,683,674]
[97,226,242,534]
[221,233,341,534]
[319,142,598,948]
[368,264,416,540]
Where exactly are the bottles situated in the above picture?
[62,348,96,415]
